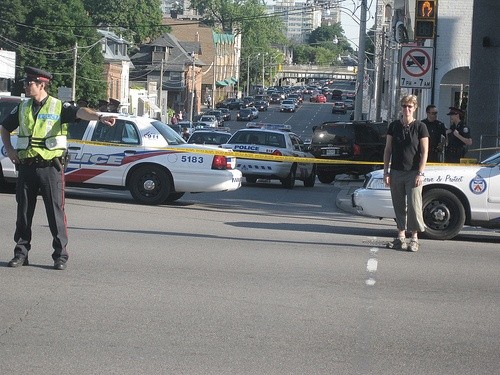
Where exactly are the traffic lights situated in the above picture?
[415,0,438,39]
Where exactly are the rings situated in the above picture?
[13,159,15,162]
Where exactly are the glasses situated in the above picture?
[428,112,437,115]
[401,105,412,108]
[23,81,35,86]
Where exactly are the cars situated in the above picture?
[0,111,244,206]
[166,77,355,140]
[187,130,233,151]
[221,128,319,190]
[0,96,33,126]
[351,153,500,240]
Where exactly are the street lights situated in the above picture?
[247,51,269,97]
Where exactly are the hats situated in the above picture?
[109,98,120,105]
[19,66,53,83]
[97,99,108,107]
[447,107,460,115]
[77,98,88,105]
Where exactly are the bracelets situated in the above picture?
[384,174,390,177]
[99,114,102,120]
[417,172,424,176]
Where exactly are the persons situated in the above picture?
[444,107,472,163]
[421,105,448,162]
[183,128,190,142]
[97,100,109,112]
[383,95,430,251]
[109,98,120,112]
[77,99,88,107]
[171,114,177,124]
[178,111,183,119]
[0,67,118,268]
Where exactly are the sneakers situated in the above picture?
[388,239,406,248]
[408,241,419,251]
[8,257,28,266]
[54,256,67,269]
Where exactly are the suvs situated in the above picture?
[309,120,388,184]
